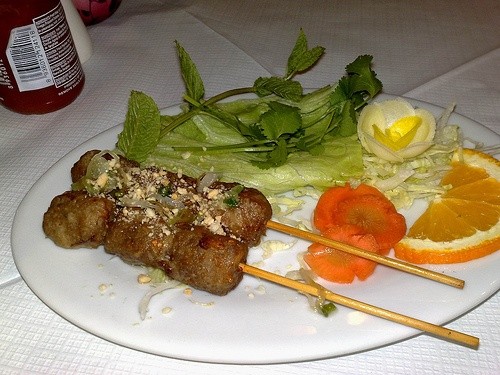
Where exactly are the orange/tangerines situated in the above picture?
[393,149,499,263]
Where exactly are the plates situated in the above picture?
[10,88,499,366]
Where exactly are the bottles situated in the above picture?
[1,0,121,115]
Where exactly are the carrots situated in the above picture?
[305,186,406,283]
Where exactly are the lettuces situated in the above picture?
[109,26,461,207]
